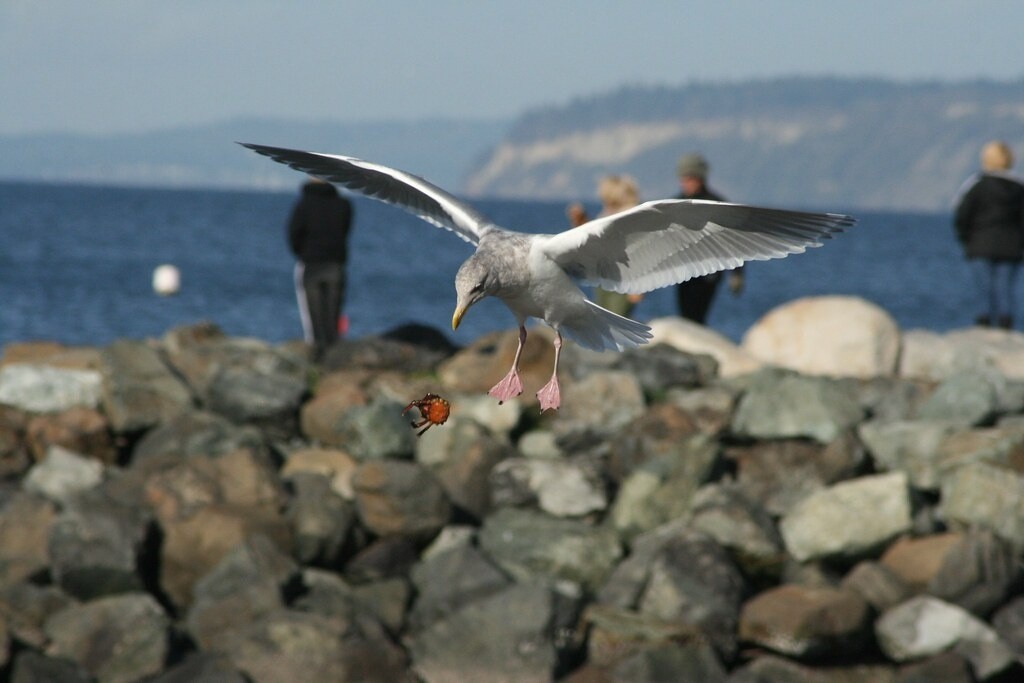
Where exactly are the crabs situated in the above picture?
[403,393,450,437]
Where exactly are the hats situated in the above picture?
[675,153,710,179]
[981,144,1011,171]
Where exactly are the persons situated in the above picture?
[568,174,645,320]
[284,175,352,360]
[954,140,1024,329]
[668,151,745,327]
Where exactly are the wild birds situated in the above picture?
[233,140,859,415]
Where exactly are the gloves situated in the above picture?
[730,273,744,297]
